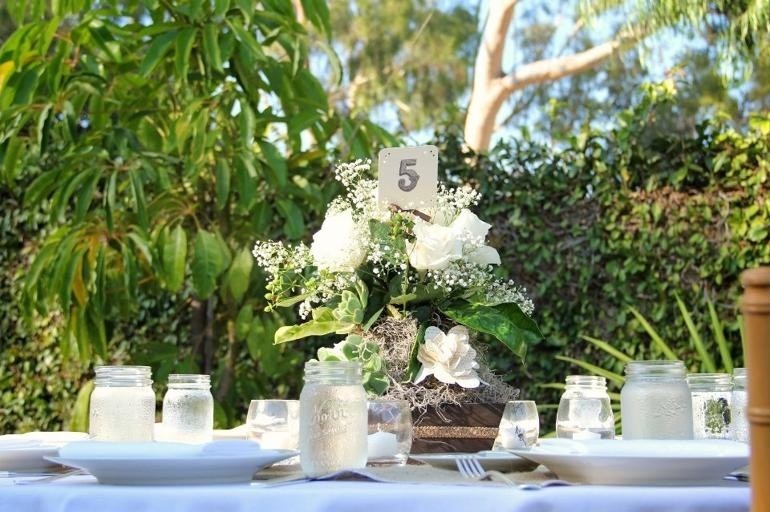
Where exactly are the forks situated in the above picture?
[454,455,540,491]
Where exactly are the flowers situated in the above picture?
[250,154,547,397]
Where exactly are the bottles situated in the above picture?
[497,399,541,452]
[620,358,696,440]
[245,398,302,452]
[686,366,750,445]
[556,373,617,441]
[162,372,216,445]
[367,399,413,470]
[87,364,157,444]
[299,360,370,481]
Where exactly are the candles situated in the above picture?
[367,420,399,461]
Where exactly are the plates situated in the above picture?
[0,430,89,474]
[505,439,752,485]
[41,452,302,487]
[408,452,541,475]
